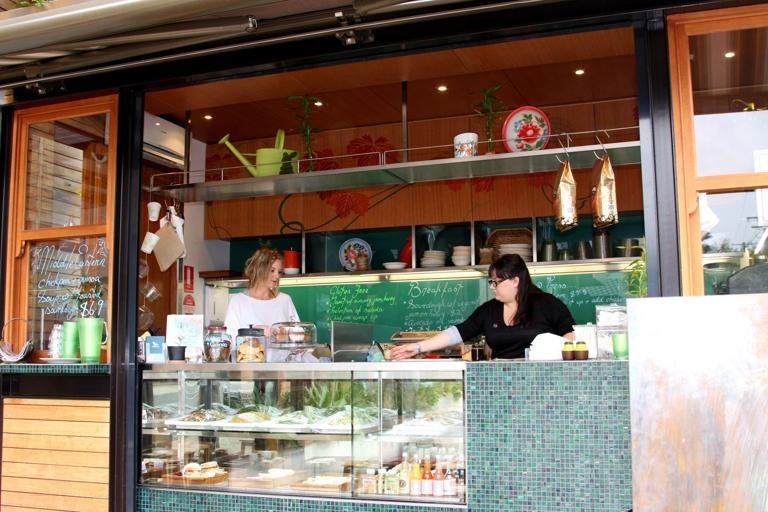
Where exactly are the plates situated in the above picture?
[420,250,447,267]
[160,410,378,436]
[497,243,532,262]
[338,237,372,271]
[501,106,552,153]
[39,358,81,365]
[383,262,409,270]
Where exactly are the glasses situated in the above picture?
[489,278,507,287]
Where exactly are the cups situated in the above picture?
[77,317,108,366]
[452,131,479,158]
[355,256,369,271]
[146,201,161,222]
[62,321,78,358]
[144,286,160,302]
[140,231,160,255]
[620,239,640,256]
[283,250,300,268]
[612,334,627,361]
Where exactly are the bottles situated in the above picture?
[561,341,588,361]
[363,444,466,506]
[592,230,610,258]
[235,322,268,363]
[540,238,592,263]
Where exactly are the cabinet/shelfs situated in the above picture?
[205,124,641,203]
[134,360,469,506]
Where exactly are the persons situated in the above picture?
[223,246,303,391]
[389,253,576,362]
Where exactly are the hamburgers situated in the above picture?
[182,460,219,477]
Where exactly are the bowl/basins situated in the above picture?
[451,245,471,265]
[282,267,299,276]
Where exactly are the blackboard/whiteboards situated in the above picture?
[227,267,646,343]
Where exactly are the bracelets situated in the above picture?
[416,342,422,354]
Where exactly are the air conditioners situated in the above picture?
[103,107,192,172]
